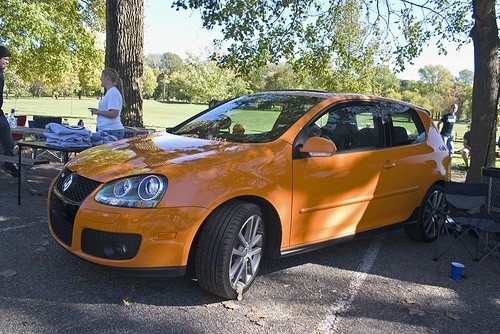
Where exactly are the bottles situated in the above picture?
[5,113,9,123]
[63,119,68,125]
[9,109,16,128]
[78,120,84,127]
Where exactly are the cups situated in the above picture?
[28,120,34,127]
[450,262,464,281]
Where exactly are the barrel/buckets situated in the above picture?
[12,116,26,139]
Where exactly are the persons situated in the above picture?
[233,124,247,135]
[462,131,472,170]
[91,68,125,139]
[294,121,322,148]
[0,45,19,178]
[437,103,458,159]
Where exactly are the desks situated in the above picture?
[9,125,47,138]
[16,141,97,205]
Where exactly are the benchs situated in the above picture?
[357,126,409,150]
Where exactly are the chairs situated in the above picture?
[433,181,500,264]
[32,115,70,163]
[330,122,360,152]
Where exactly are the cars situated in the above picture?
[47,88,452,299]
